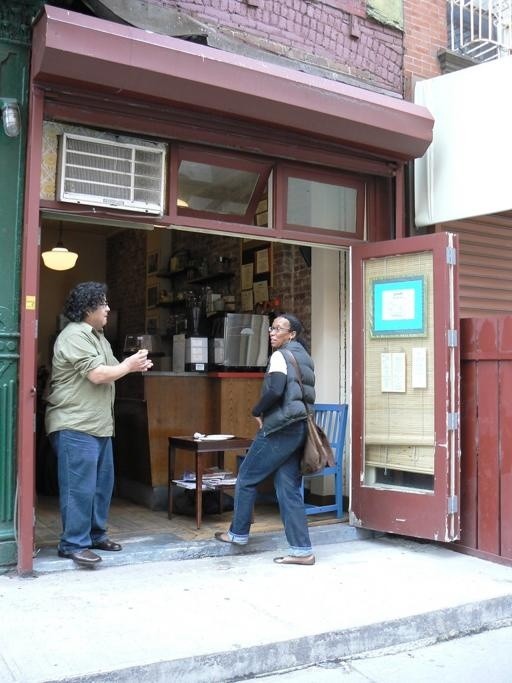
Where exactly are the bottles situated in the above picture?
[161,252,233,335]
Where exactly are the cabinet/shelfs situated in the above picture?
[162,262,233,338]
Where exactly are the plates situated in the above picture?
[199,433,235,441]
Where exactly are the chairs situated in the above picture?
[281,401,352,524]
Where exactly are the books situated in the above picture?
[171,463,238,490]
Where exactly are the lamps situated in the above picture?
[1,97,23,136]
[41,220,78,271]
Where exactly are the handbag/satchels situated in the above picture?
[299,412,337,475]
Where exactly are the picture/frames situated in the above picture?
[144,248,161,276]
[145,315,161,334]
[145,283,160,309]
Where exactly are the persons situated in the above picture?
[42,279,154,565]
[211,312,316,566]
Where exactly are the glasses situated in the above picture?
[98,301,109,309]
[268,326,293,332]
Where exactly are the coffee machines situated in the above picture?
[207,312,269,370]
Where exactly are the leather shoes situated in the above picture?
[273,552,317,565]
[214,530,249,547]
[88,536,122,551]
[57,542,101,565]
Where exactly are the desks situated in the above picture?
[169,432,256,527]
